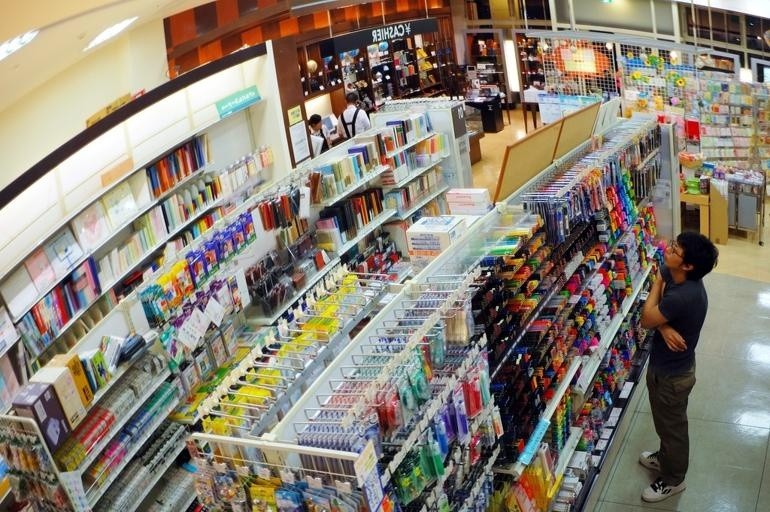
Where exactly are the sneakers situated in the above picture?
[639,450,662,471]
[641,476,687,503]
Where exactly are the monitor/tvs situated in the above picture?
[467,66,476,71]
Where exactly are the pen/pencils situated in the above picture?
[458,399,468,434]
[438,418,449,454]
[432,441,445,475]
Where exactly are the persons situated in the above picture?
[578,68,620,97]
[305,92,370,154]
[638,231,718,503]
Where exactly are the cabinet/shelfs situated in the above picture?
[0,16,681,512]
[728,192,758,232]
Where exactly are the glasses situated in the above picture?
[670,239,682,260]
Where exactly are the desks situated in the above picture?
[680,192,710,239]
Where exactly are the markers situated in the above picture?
[558,210,566,243]
[563,207,570,236]
